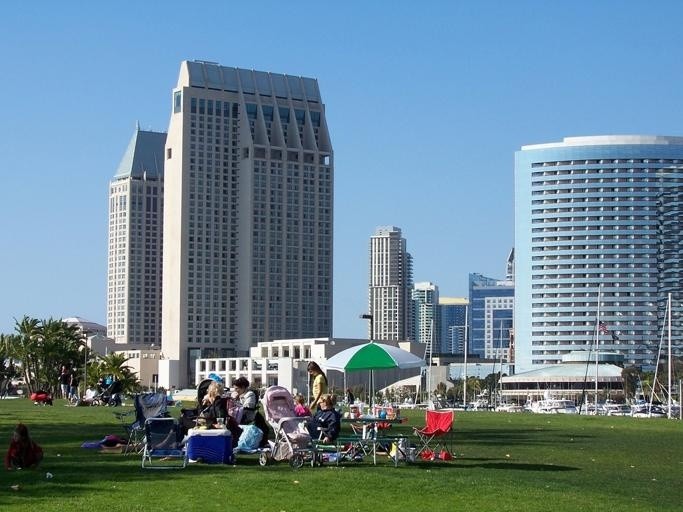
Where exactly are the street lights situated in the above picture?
[360,315,373,410]
[82,329,90,397]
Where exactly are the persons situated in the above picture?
[4,423,43,470]
[31,365,114,406]
[153,361,341,461]
[345,388,355,406]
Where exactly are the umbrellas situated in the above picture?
[318,340,426,417]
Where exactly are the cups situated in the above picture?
[216,417,224,428]
[226,417,229,424]
[328,453,334,462]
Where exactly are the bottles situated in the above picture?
[348,397,400,420]
[362,421,378,440]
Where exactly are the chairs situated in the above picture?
[123,386,455,470]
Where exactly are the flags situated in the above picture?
[599,320,607,333]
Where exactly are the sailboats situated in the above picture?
[376,283,683,420]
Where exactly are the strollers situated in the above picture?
[92,380,123,407]
[258,385,325,469]
[197,376,241,447]
[30,384,55,407]
